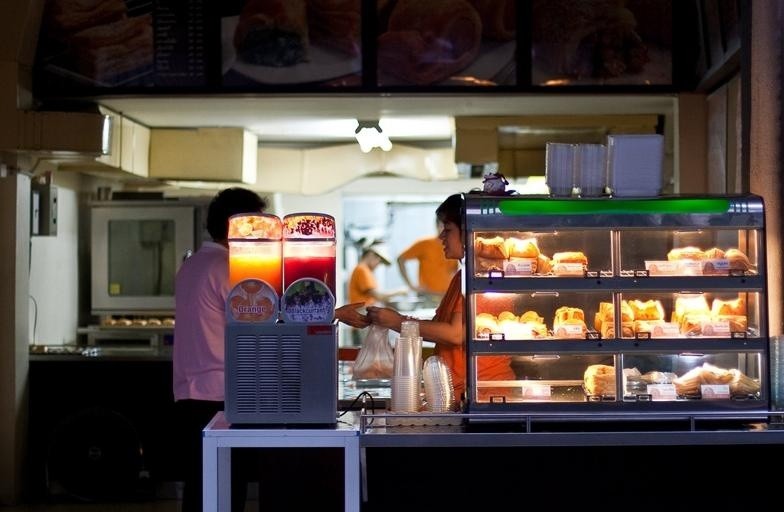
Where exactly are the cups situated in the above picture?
[390,321,422,414]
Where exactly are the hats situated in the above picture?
[368,242,392,265]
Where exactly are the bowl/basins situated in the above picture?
[423,355,456,412]
[545,143,608,197]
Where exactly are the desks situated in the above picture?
[360,424,783,501]
[202,410,360,512]
[27,347,173,504]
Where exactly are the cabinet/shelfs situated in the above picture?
[463,194,773,422]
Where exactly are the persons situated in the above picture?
[396,217,460,293]
[173,188,370,512]
[347,240,390,346]
[366,191,516,399]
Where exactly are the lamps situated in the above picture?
[356,118,393,152]
